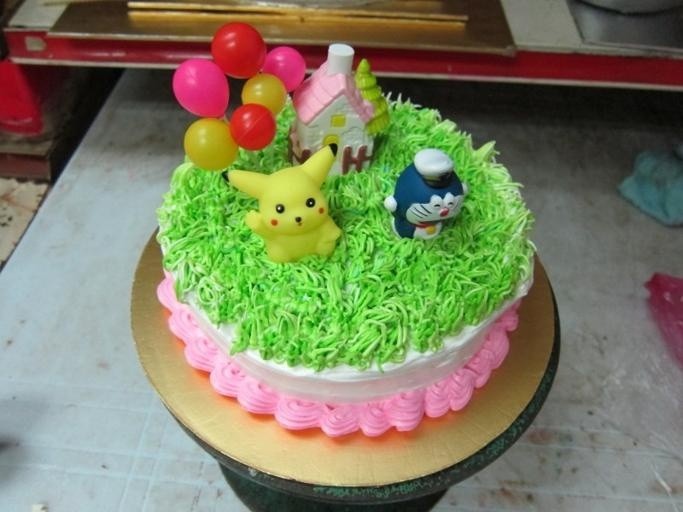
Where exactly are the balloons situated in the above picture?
[171,21,306,172]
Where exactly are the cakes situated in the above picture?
[156,43,538,438]
